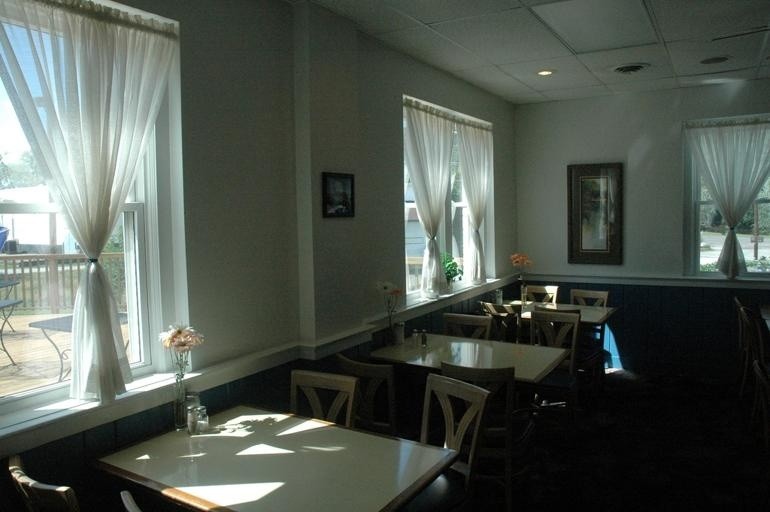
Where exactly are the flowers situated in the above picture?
[156,318,205,429]
[376,278,407,326]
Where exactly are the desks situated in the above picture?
[93,402,461,512]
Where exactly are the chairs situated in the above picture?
[398,374,491,511]
[439,359,515,441]
[730,290,770,431]
[478,281,617,392]
[440,311,493,340]
[7,454,84,511]
[336,352,397,436]
[290,369,359,424]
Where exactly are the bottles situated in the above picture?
[495,289,502,304]
[186,405,198,435]
[419,329,428,348]
[411,328,420,348]
[197,406,210,434]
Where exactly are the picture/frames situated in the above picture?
[319,170,356,218]
[567,162,624,265]
